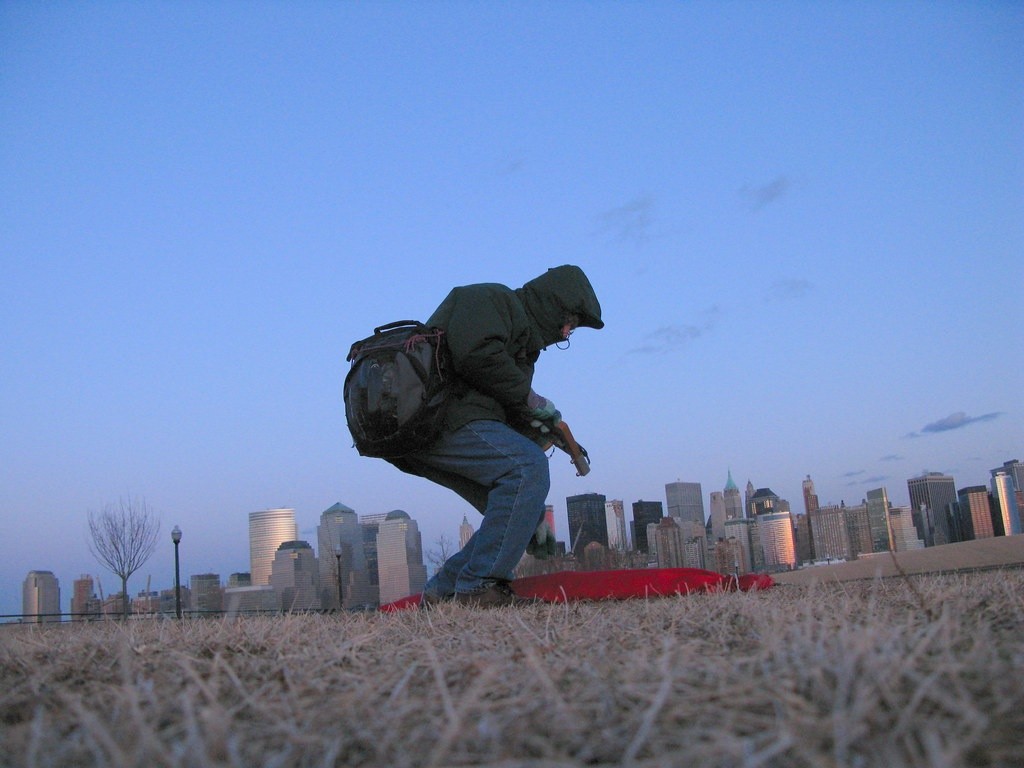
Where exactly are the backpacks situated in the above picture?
[343,320,453,457]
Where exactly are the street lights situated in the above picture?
[169,524,183,620]
[334,543,343,612]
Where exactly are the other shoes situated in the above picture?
[420,593,455,608]
[455,580,544,611]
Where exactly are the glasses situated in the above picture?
[564,318,575,334]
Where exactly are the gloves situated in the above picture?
[524,389,562,428]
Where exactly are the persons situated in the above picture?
[378,265,608,609]
